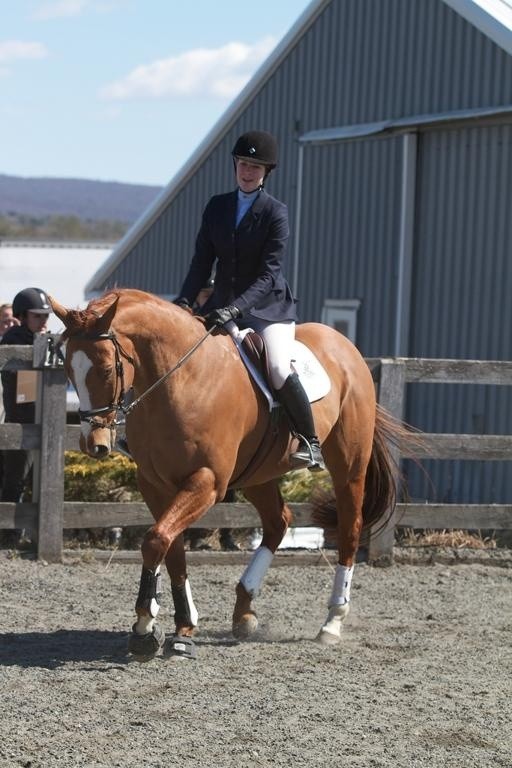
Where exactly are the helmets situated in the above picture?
[232,131,276,166]
[12,288,53,317]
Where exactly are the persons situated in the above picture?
[172,130,325,472]
[0,288,51,548]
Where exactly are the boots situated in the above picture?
[275,373,325,472]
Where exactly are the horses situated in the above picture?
[43,281,443,666]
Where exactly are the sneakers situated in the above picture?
[190,538,212,551]
[220,535,239,551]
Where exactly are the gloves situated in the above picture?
[204,306,240,326]
